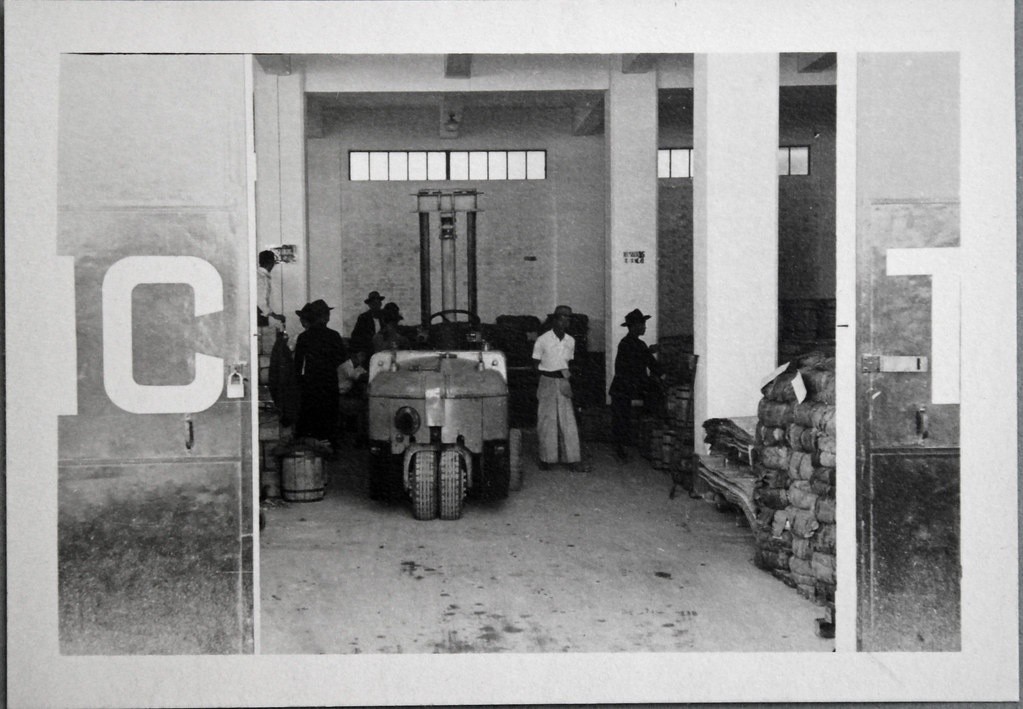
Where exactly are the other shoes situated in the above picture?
[571,461,591,471]
[538,462,553,470]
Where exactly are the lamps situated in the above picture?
[444,113,461,130]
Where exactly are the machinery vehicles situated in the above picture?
[365,309,543,523]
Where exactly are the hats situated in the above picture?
[620,309,651,327]
[374,303,404,320]
[365,291,385,304]
[296,299,334,321]
[547,306,579,324]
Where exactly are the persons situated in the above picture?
[336,341,378,448]
[292,299,349,441]
[531,305,591,473]
[351,291,386,367]
[257,250,286,334]
[372,302,412,352]
[608,309,668,462]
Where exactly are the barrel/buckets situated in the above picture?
[281,451,326,501]
[578,387,695,472]
